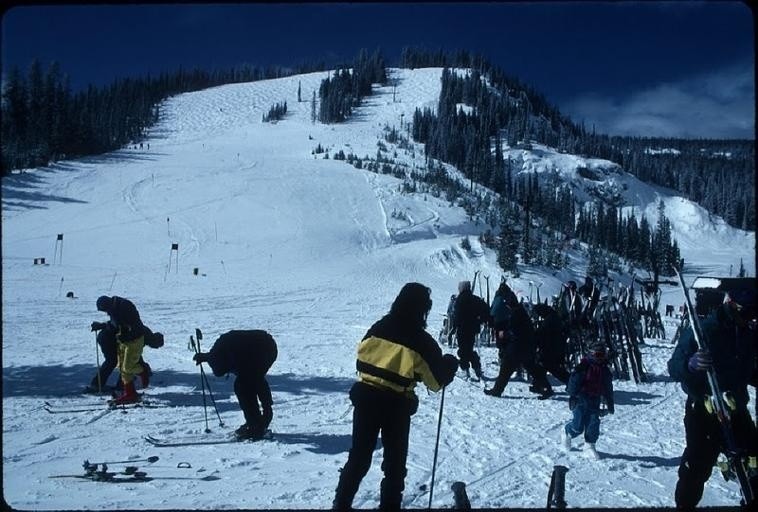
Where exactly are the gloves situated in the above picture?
[569,396,578,410]
[689,349,715,375]
[608,401,615,414]
[91,322,104,332]
[443,354,459,377]
[193,351,211,365]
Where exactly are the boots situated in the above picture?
[456,369,470,378]
[561,425,572,450]
[474,367,489,382]
[136,366,148,389]
[583,442,600,460]
[116,382,137,403]
[234,415,259,435]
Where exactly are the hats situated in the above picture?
[96,296,113,311]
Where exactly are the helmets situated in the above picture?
[729,286,758,314]
[588,343,607,357]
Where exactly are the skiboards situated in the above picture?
[444,271,666,383]
[42,398,168,412]
[145,430,274,445]
[673,257,754,508]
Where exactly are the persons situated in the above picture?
[561,343,615,462]
[329,281,460,511]
[664,277,758,506]
[444,277,627,400]
[192,329,279,439]
[89,318,165,390]
[89,292,152,406]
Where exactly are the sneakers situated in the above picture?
[538,390,556,400]
[484,389,502,398]
